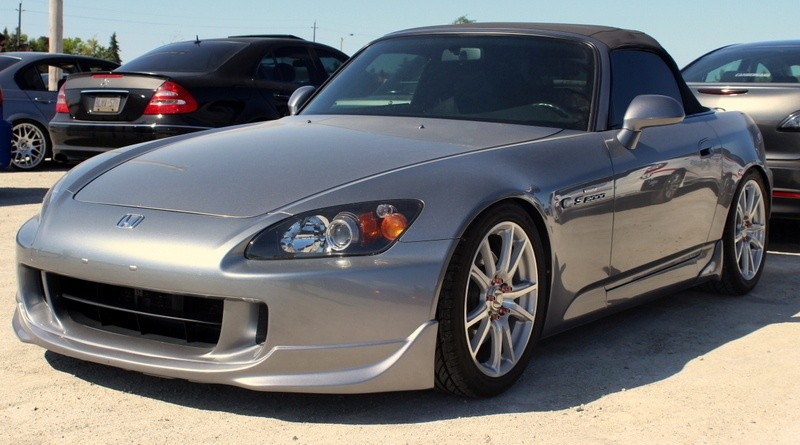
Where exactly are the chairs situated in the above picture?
[270,63,296,83]
[720,71,753,82]
[57,79,66,92]
[770,76,798,83]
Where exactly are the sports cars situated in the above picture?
[13,22,775,404]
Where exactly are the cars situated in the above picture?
[0,51,121,172]
[675,38,800,224]
[48,34,404,165]
